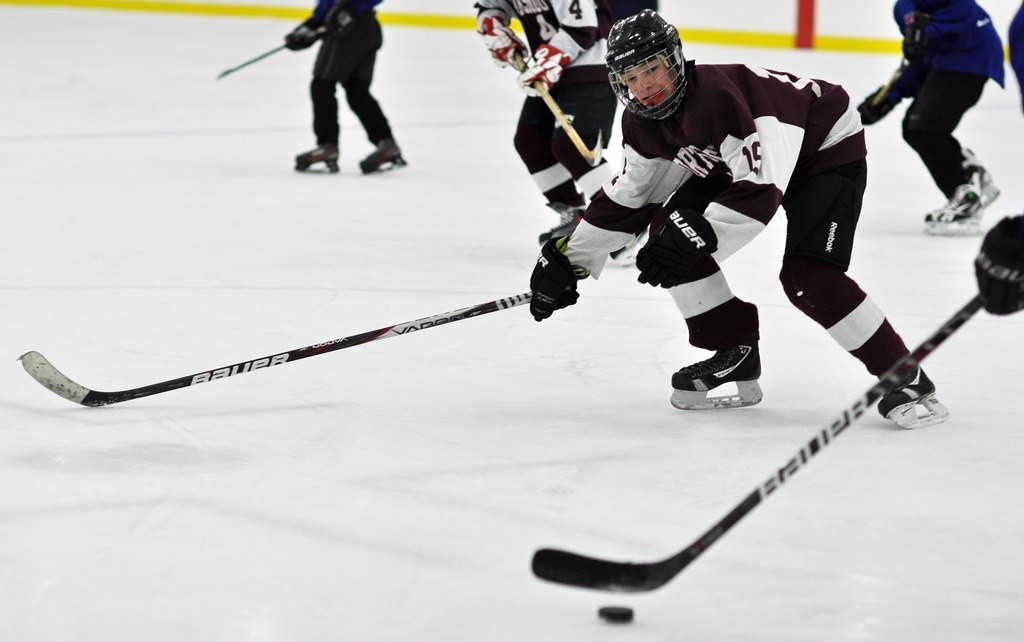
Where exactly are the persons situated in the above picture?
[530,9,936,417]
[856,0,1005,223]
[285,0,400,169]
[974,4,1024,315]
[474,0,647,259]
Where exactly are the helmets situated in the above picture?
[603,8,689,121]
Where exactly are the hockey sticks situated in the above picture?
[532,294,985,594]
[19,278,529,407]
[216,30,322,79]
[512,50,604,167]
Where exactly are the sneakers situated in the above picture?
[536,193,587,243]
[360,137,406,173]
[295,144,341,173]
[924,148,1001,237]
[610,227,649,269]
[877,364,950,429]
[670,338,764,411]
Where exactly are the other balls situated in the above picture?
[598,607,632,621]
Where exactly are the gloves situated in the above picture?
[974,214,1024,315]
[637,210,720,289]
[480,14,529,67]
[516,42,571,96]
[529,235,580,322]
[323,1,370,34]
[858,84,903,125]
[901,25,930,64]
[283,15,328,50]
